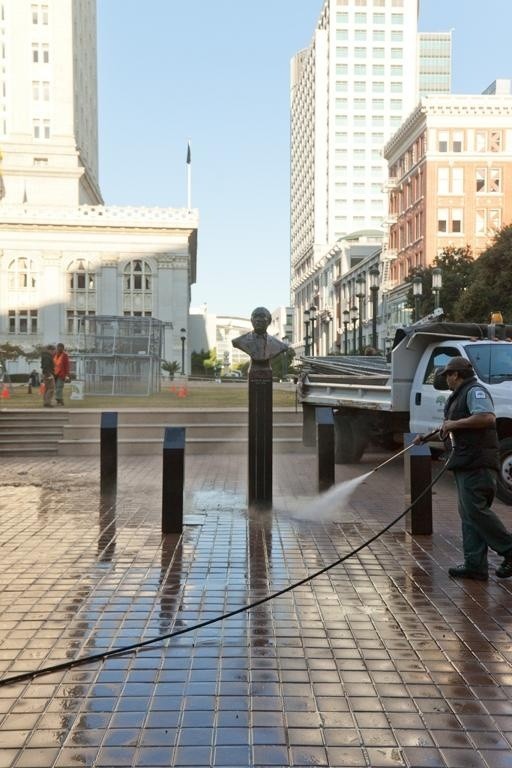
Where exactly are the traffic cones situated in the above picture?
[0,383,10,399]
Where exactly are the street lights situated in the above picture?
[412,268,443,321]
[281,336,289,379]
[343,269,380,355]
[303,303,317,357]
[180,328,186,375]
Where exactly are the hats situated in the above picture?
[438,356,473,376]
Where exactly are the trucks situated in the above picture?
[295,321,512,505]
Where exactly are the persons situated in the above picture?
[232,305,289,380]
[52,341,72,406]
[41,344,58,408]
[410,355,511,581]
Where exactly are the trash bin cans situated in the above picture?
[28,372,39,387]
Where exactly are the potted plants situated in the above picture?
[160,357,181,382]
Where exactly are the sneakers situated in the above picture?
[449,564,489,581]
[496,558,512,578]
